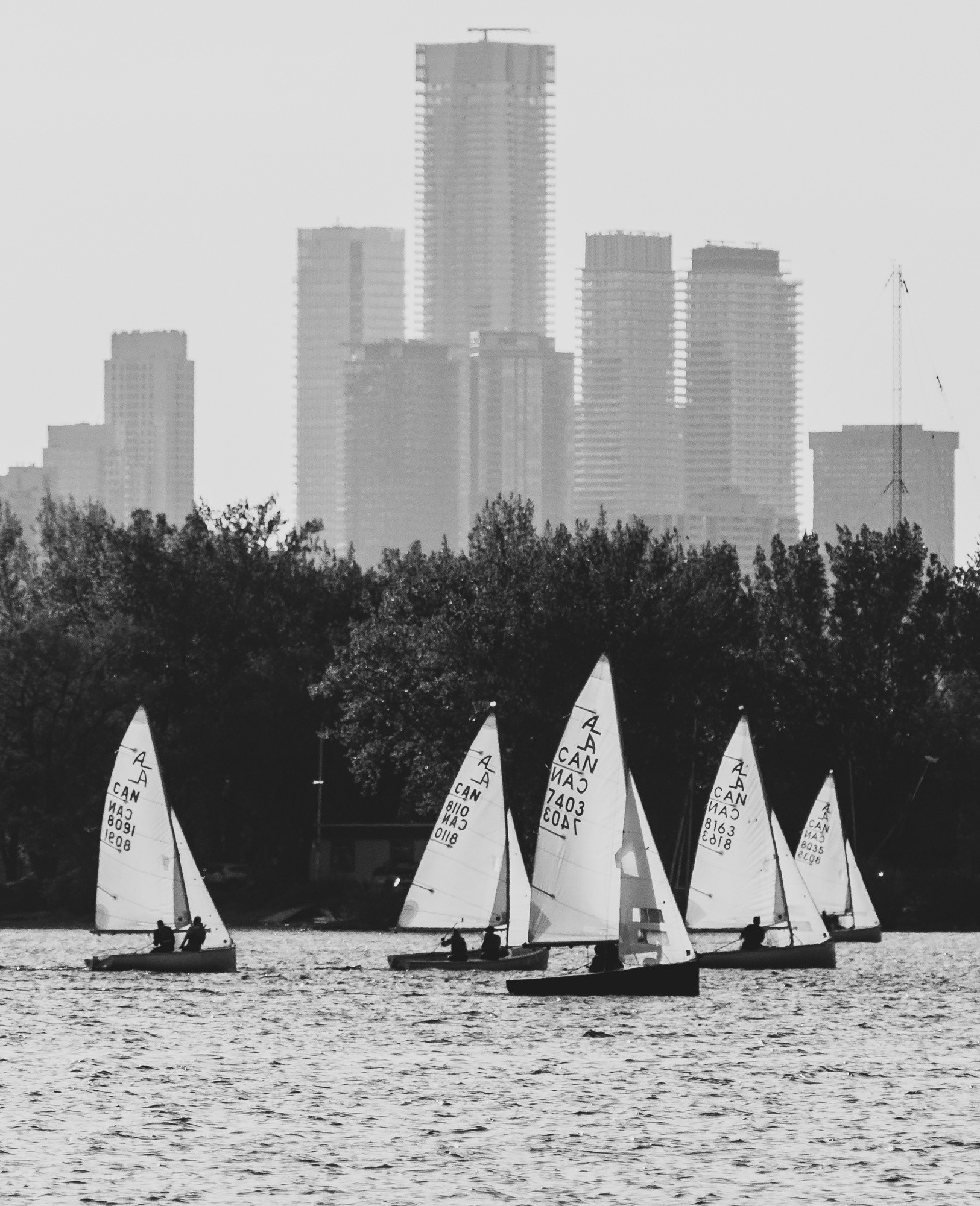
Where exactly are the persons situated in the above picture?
[739,917,765,950]
[481,926,500,961]
[441,929,468,962]
[149,920,176,953]
[179,916,206,951]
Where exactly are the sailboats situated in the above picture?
[507,654,705,999]
[80,705,240,973]
[792,769,887,946]
[374,700,561,975]
[680,707,843,974]
